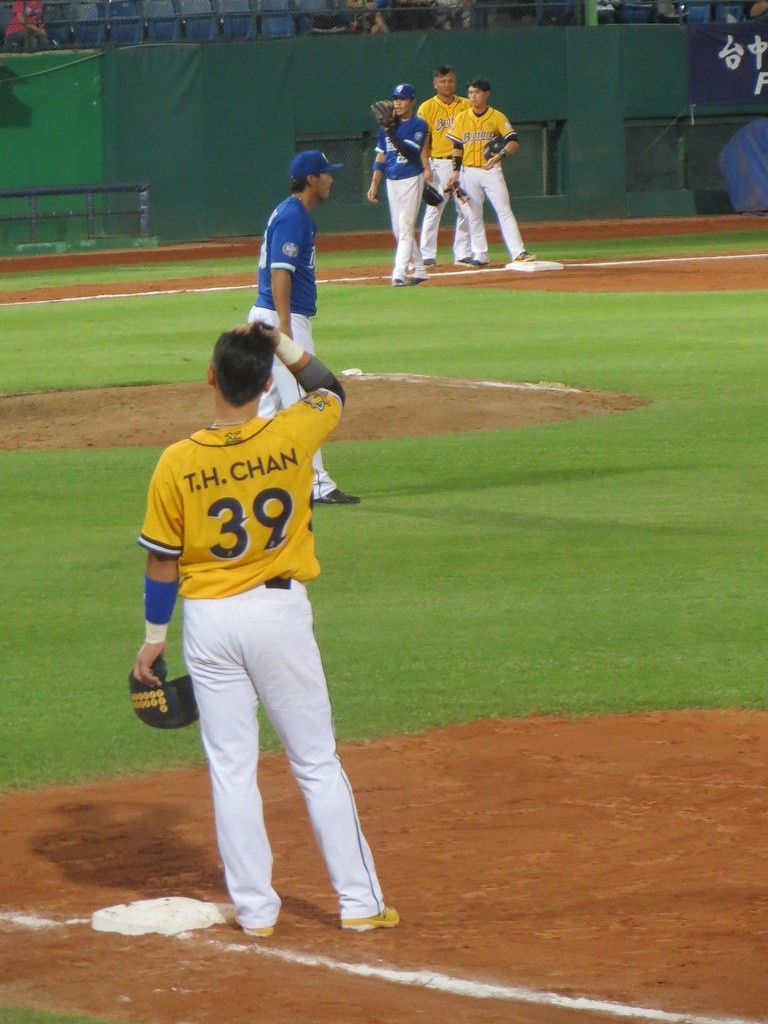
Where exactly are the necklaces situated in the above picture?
[210,417,250,426]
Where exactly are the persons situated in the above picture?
[417,66,473,268]
[346,0,768,35]
[246,151,362,505]
[445,78,537,268]
[133,319,401,934]
[367,83,430,287]
[4,0,51,51]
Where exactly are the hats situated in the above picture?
[392,83,416,98]
[290,149,343,178]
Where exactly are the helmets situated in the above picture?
[129,657,201,730]
[422,183,444,206]
[484,135,506,160]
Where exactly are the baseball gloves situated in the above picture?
[370,99,398,132]
[423,183,444,206]
[128,656,200,731]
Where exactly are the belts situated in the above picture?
[265,577,291,588]
[437,156,453,159]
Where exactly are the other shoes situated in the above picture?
[409,277,426,285]
[394,280,405,286]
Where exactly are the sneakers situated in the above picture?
[466,259,489,267]
[234,913,274,937]
[424,259,436,267]
[512,252,536,263]
[455,257,472,265]
[341,904,400,931]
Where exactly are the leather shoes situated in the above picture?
[313,488,360,503]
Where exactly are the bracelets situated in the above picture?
[145,620,167,643]
[498,147,510,160]
[372,161,386,173]
[274,332,305,367]
